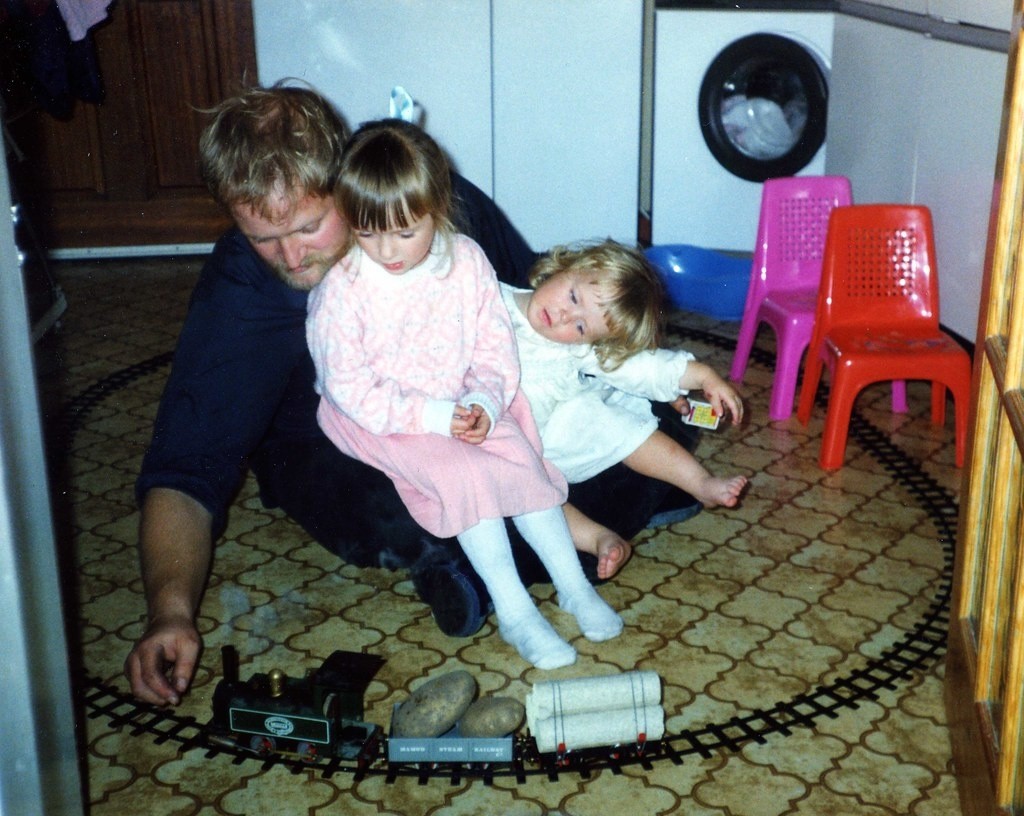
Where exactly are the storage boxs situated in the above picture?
[388,703,513,761]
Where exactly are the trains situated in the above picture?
[211,645,666,769]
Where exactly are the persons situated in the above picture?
[304,119,623,672]
[123,77,701,707]
[498,236,748,582]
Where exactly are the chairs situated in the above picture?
[730,177,909,419]
[796,204,973,470]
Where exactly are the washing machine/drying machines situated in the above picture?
[641,1,836,250]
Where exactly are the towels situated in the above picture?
[524,666,667,753]
[56,0,112,42]
